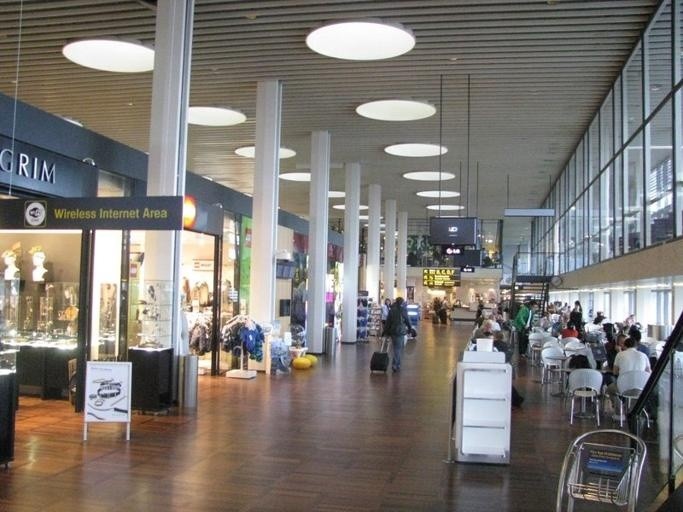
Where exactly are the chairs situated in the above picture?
[529,312,651,429]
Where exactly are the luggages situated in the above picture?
[370,337,390,375]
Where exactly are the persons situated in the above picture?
[471,309,509,361]
[440,296,449,325]
[536,301,652,423]
[380,297,412,375]
[474,298,483,327]
[513,300,538,361]
[381,298,390,339]
[481,248,491,264]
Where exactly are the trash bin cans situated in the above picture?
[325,327,336,360]
[178,355,198,408]
[0,370,17,471]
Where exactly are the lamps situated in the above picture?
[327,190,346,200]
[381,141,450,160]
[278,170,316,184]
[414,189,461,199]
[63,33,155,76]
[426,204,465,213]
[233,143,298,164]
[353,96,439,123]
[187,102,247,129]
[332,203,369,213]
[301,21,417,63]
[402,170,456,183]
[359,214,398,234]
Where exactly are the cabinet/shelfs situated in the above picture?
[0,278,80,468]
[367,307,382,344]
[127,279,172,416]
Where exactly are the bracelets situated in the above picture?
[97,385,120,398]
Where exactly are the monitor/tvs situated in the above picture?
[453,250,482,266]
[442,246,464,255]
[429,217,476,246]
